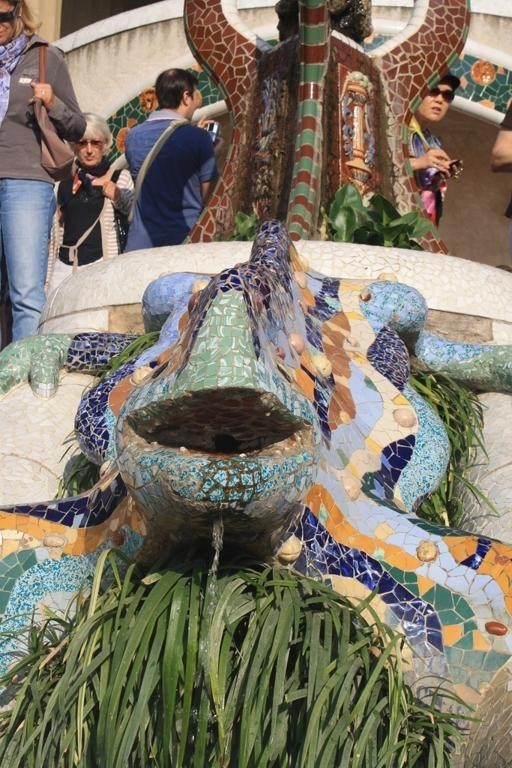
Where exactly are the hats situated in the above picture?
[441,71,461,91]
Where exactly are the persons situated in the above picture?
[488,100,512,220]
[49,113,137,268]
[124,67,219,257]
[400,61,464,229]
[1,0,87,343]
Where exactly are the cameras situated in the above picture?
[206,121,221,143]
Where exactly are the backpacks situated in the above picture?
[27,43,76,182]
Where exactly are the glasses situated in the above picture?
[0,0,21,22]
[76,140,106,148]
[430,87,455,101]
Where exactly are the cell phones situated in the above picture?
[434,159,460,177]
